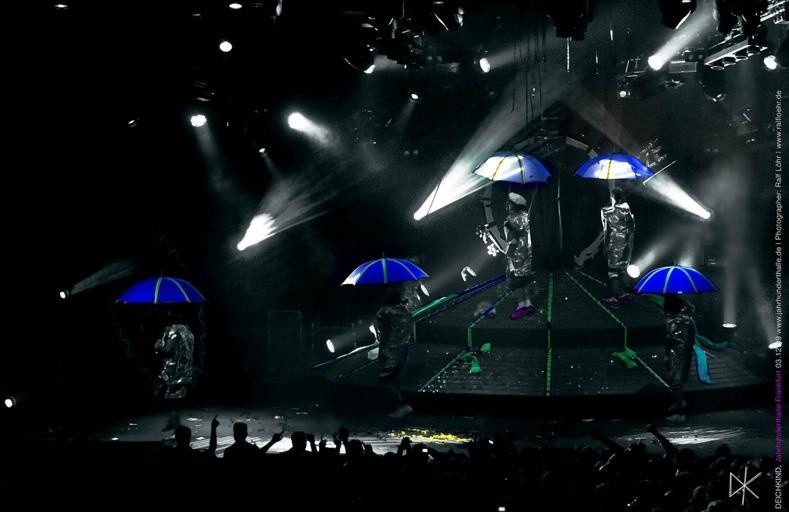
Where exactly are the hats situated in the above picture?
[508,192,527,206]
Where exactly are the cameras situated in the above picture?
[496,505,507,512]
[421,448,429,454]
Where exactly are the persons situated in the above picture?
[601,188,636,309]
[154,312,195,432]
[376,287,414,417]
[504,192,536,320]
[163,415,789,512]
[664,296,694,422]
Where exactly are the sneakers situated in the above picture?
[509,304,536,320]
[603,294,634,310]
[666,414,686,423]
[387,404,413,419]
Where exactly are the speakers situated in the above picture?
[266,311,304,377]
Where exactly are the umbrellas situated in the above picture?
[472,151,552,192]
[633,259,720,297]
[573,153,654,188]
[115,276,207,304]
[340,257,431,287]
